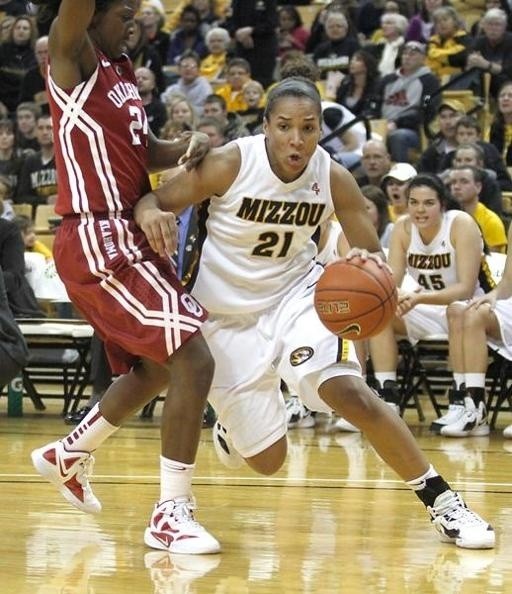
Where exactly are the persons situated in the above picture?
[30,1,221,554]
[0,1,511,442]
[131,59,497,549]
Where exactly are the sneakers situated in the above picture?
[213,420,242,470]
[30,439,103,514]
[425,490,495,549]
[145,495,220,554]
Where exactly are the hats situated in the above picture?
[378,162,418,184]
[438,98,466,115]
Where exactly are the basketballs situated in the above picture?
[314,255,397,341]
